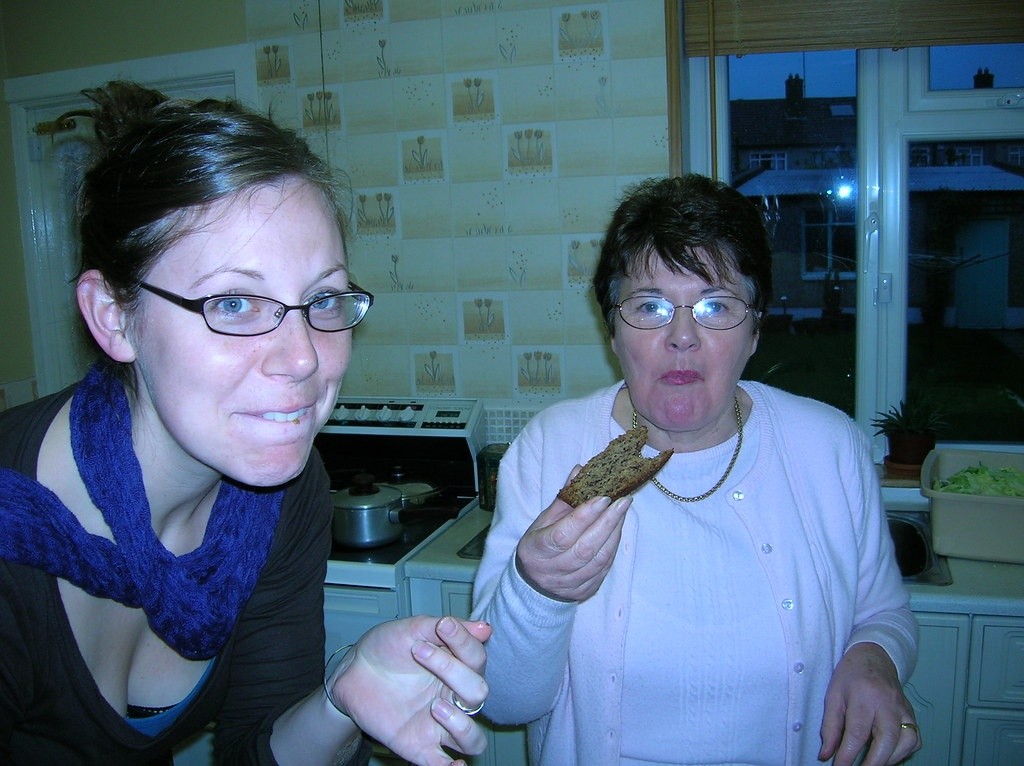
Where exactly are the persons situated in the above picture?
[470,174,922,766]
[0,80,494,766]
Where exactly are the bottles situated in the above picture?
[476,443,510,510]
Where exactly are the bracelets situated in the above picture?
[321,645,357,717]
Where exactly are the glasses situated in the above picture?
[616,295,755,331]
[138,276,374,338]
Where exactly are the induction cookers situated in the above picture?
[315,396,487,563]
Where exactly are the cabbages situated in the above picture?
[931,458,1024,499]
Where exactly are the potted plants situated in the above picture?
[869,396,951,479]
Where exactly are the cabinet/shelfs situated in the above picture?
[167,577,1024,766]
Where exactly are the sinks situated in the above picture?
[456,520,491,562]
[882,508,954,585]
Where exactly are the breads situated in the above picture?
[556,425,674,507]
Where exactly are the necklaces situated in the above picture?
[632,393,743,503]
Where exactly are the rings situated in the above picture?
[452,689,486,717]
[901,723,917,732]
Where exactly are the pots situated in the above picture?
[332,474,460,549]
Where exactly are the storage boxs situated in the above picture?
[921,448,1024,565]
